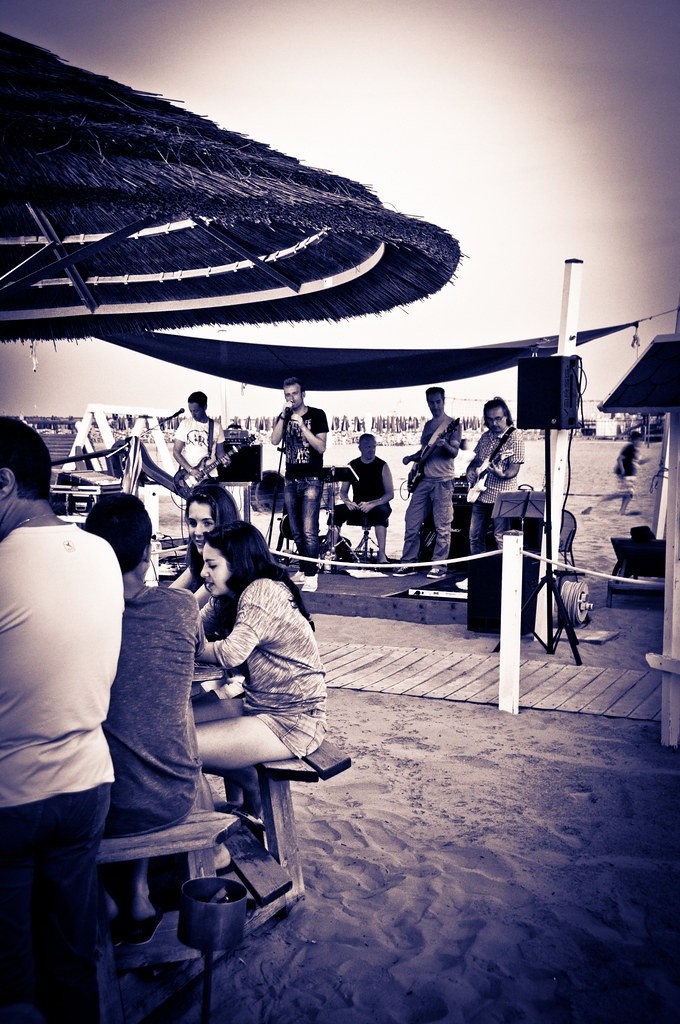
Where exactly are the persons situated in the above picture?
[392,387,462,579]
[0,417,127,1024]
[598,432,643,516]
[85,485,328,947]
[270,377,329,593]
[456,399,526,591]
[327,433,394,564]
[172,391,232,568]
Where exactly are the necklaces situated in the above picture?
[15,513,56,527]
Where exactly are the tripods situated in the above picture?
[492,430,582,665]
[319,465,361,569]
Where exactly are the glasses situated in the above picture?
[486,415,505,422]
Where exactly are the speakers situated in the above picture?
[467,550,540,636]
[516,356,579,430]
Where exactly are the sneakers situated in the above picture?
[289,569,318,592]
[426,568,446,579]
[392,567,417,576]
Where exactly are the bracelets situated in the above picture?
[279,416,286,420]
[189,468,194,474]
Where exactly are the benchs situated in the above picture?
[94,740,352,973]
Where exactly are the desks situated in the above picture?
[184,661,222,878]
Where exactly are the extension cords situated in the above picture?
[408,589,468,599]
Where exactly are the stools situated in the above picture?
[347,518,378,565]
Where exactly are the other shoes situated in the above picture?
[455,578,468,590]
[216,858,236,878]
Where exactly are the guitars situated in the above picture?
[466,425,515,504]
[172,433,257,500]
[407,417,460,492]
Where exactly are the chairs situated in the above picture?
[558,509,578,582]
[86,426,540,446]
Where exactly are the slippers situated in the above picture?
[106,900,164,947]
[228,806,266,830]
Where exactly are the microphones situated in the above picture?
[286,400,294,412]
[169,408,184,419]
